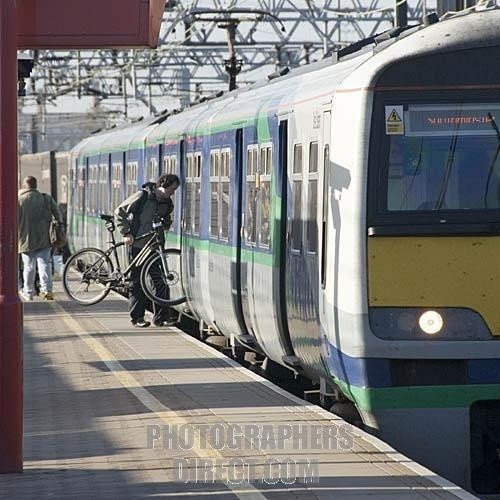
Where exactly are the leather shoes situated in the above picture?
[134,320,150,328]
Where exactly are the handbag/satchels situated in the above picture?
[48,222,66,248]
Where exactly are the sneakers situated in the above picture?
[154,320,178,326]
[20,289,54,301]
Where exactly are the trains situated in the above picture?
[20,4,500,498]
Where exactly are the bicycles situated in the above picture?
[62,214,187,307]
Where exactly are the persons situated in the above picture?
[113,173,182,329]
[17,175,64,301]
